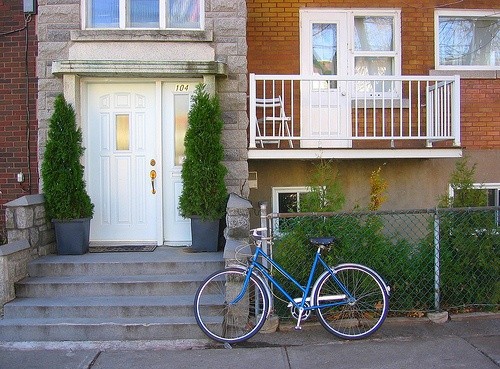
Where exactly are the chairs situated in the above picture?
[256,95,295,149]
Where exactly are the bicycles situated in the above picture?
[193,229,391,344]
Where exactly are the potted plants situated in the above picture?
[41,92,96,256]
[177,82,230,253]
